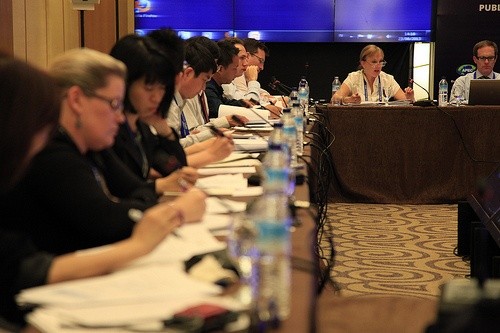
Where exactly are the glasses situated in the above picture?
[92,95,124,110]
[477,55,496,61]
[251,53,265,64]
[365,60,387,69]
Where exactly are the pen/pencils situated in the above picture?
[128,210,187,244]
[210,130,219,138]
[176,176,208,200]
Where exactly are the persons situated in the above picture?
[146,26,186,175]
[220,37,260,102]
[232,38,290,109]
[0,48,184,291]
[187,37,249,131]
[331,45,415,104]
[149,42,235,147]
[90,33,199,201]
[449,41,500,104]
[205,41,282,119]
[22,49,206,257]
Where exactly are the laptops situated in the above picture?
[453,79,500,104]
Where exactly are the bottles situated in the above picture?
[439,76,448,107]
[332,76,341,106]
[252,79,309,322]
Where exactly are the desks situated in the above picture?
[315,100,500,204]
[23,94,317,333]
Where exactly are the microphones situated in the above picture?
[242,102,276,128]
[230,115,267,141]
[408,77,433,106]
[249,98,282,119]
[209,126,258,160]
[268,78,299,108]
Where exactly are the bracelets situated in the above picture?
[166,133,173,137]
[340,96,346,105]
